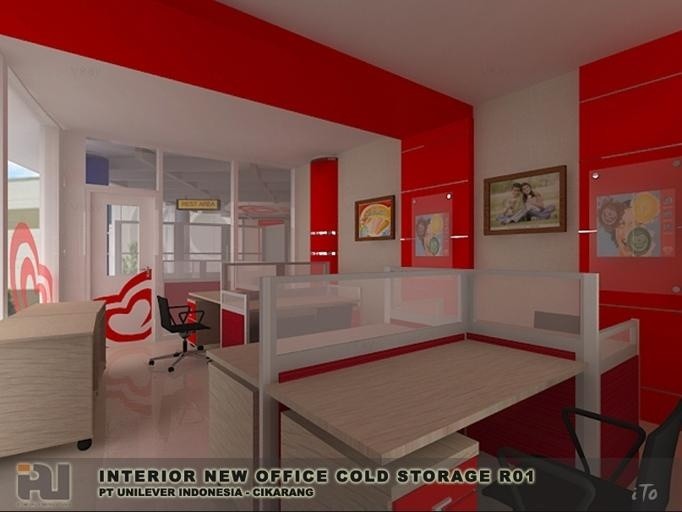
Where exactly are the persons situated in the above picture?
[495,181,527,223]
[518,182,556,221]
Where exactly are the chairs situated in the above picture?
[148,295,211,373]
[483,399,681,511]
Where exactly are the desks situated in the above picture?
[189,290,586,512]
[0,300,107,459]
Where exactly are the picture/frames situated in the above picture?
[354,195,395,241]
[484,165,567,234]
